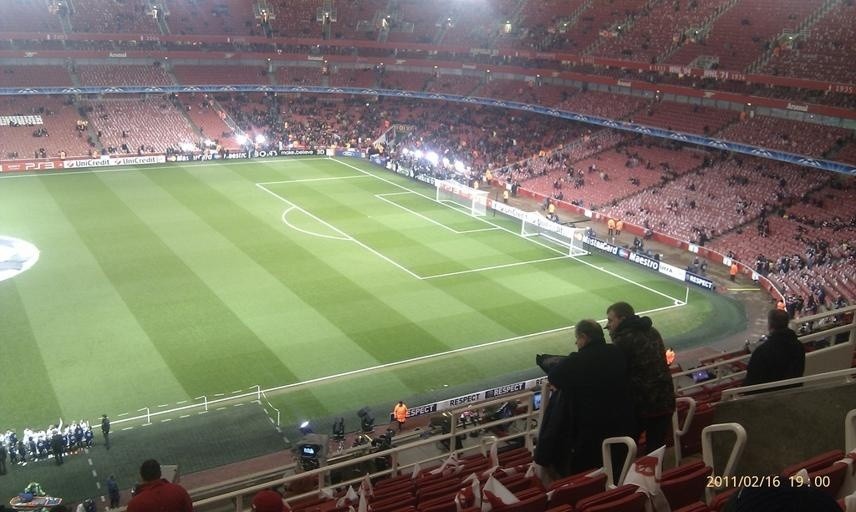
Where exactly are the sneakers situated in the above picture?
[78,446,92,451]
[10,458,18,464]
[70,451,78,455]
[30,457,46,462]
[17,461,28,467]
[104,444,110,450]
[48,451,68,459]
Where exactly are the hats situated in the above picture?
[141,460,161,477]
[25,482,45,496]
[251,490,282,511]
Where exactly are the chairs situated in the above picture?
[2,0,856,255]
[114,255,856,511]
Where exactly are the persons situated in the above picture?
[664,347,675,366]
[0,1,855,185]
[740,309,806,395]
[0,417,95,476]
[126,459,192,512]
[459,404,521,442]
[107,474,120,509]
[75,496,97,512]
[532,318,634,482]
[250,487,292,512]
[393,399,407,431]
[604,302,678,458]
[470,184,855,344]
[101,414,111,449]
